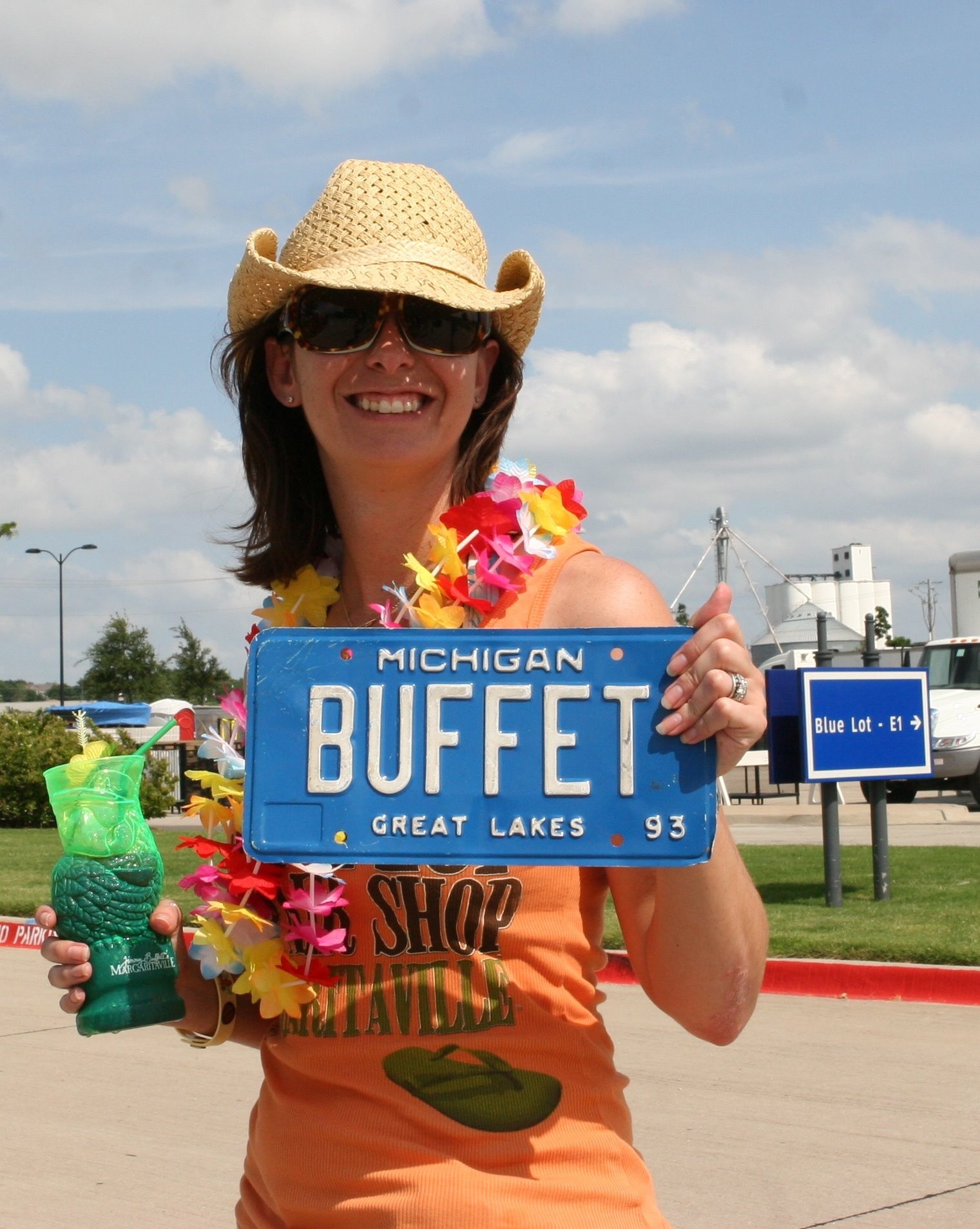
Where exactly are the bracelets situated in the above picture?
[176,970,237,1050]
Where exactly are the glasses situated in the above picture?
[280,283,495,355]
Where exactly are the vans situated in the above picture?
[860,637,980,810]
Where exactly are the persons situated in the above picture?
[32,159,769,1228]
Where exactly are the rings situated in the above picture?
[728,671,747,701]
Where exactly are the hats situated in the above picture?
[228,160,545,358]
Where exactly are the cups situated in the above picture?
[42,759,187,1038]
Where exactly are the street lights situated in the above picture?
[25,543,98,704]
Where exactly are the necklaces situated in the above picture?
[175,460,584,1018]
[363,587,419,629]
